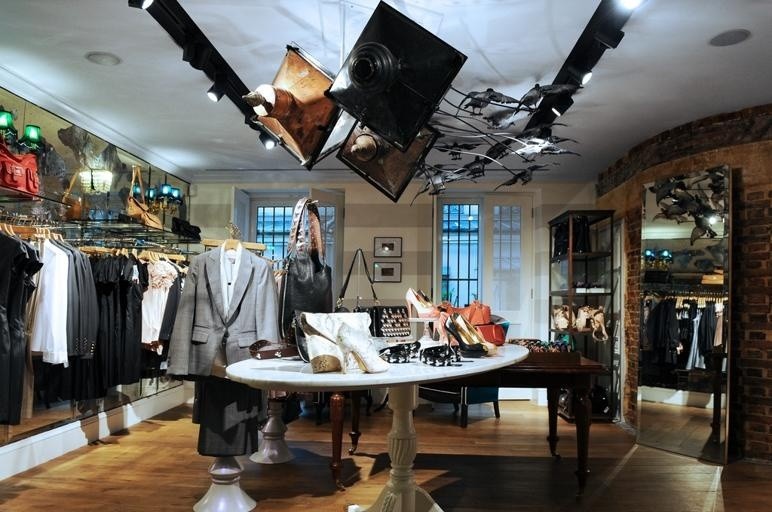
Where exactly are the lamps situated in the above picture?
[512,0,642,142]
[18,125,47,148]
[160,184,181,211]
[78,172,95,194]
[91,170,114,194]
[133,182,156,201]
[0,104,20,137]
[128,0,280,150]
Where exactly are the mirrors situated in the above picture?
[635,163,731,464]
[0,86,191,448]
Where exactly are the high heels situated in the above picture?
[406,288,440,319]
[444,313,497,358]
[337,323,389,374]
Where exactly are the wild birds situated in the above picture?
[647,164,729,246]
[410,84,585,208]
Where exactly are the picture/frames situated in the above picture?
[373,262,401,282]
[374,237,402,257]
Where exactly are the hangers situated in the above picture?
[0,207,63,241]
[77,245,185,266]
[640,288,728,310]
[223,223,239,252]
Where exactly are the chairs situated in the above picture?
[374,315,511,428]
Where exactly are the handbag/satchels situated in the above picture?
[554,215,591,257]
[575,306,609,342]
[278,250,333,345]
[442,300,492,326]
[0,144,39,194]
[551,305,576,331]
[63,199,91,221]
[371,305,411,337]
[126,199,148,217]
[301,312,372,372]
[558,378,611,416]
[144,214,162,229]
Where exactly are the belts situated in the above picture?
[378,343,421,363]
[420,345,461,366]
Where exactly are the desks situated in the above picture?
[226,340,531,512]
[329,349,610,501]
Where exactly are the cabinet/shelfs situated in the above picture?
[547,209,617,423]
